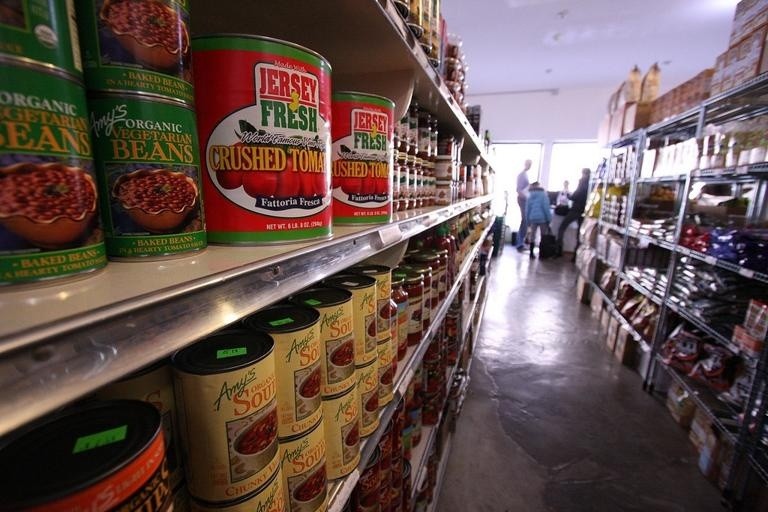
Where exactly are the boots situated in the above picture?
[530,241,536,259]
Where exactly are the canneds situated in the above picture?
[0,0,489,288]
[0,205,490,511]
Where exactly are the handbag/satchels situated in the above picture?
[539,225,558,260]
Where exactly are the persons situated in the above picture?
[555,168,590,263]
[556,180,570,207]
[525,182,552,259]
[516,159,532,250]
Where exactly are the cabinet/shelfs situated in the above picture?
[0,0,497,512]
[576,73,768,512]
